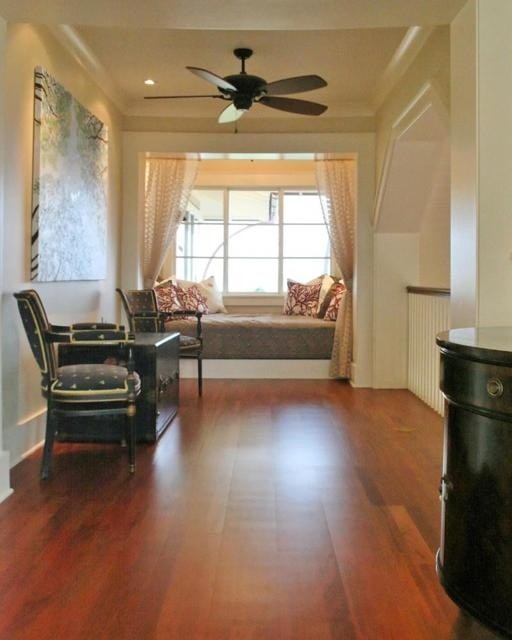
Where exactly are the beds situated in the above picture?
[163,309,340,361]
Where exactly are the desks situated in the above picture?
[56,332,181,442]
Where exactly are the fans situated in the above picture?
[142,46,331,124]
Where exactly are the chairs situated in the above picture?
[14,288,143,482]
[114,286,204,398]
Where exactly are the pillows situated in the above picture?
[281,273,347,321]
[144,273,227,313]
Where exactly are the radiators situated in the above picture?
[405,290,457,417]
